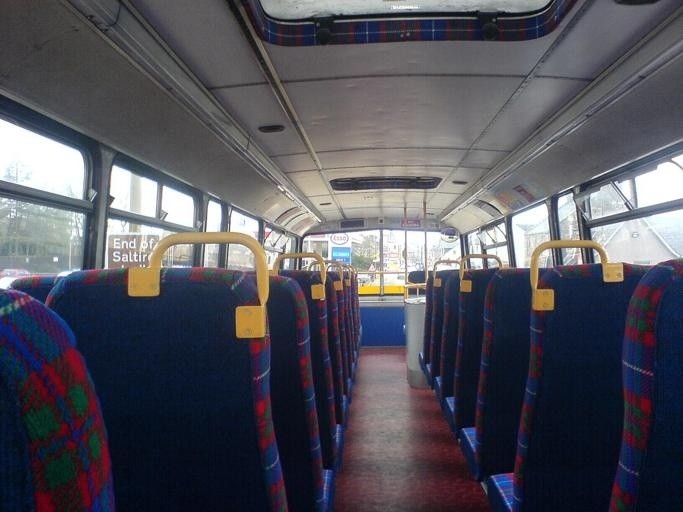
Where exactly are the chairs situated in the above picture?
[0,230,362,512]
[409,239,683,511]
